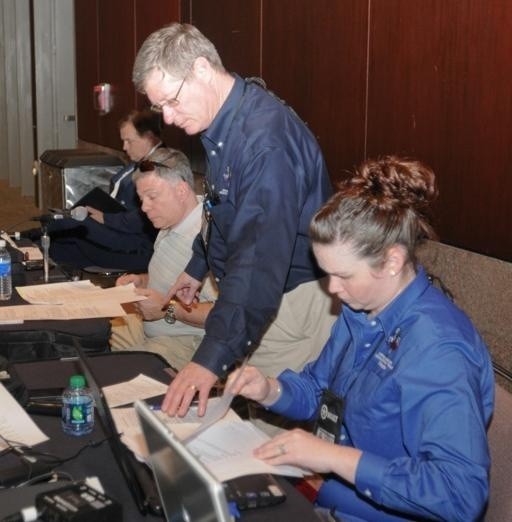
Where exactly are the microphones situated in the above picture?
[30,206,88,221]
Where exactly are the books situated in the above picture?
[48,185,128,219]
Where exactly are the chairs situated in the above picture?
[413,237,511,521]
[80,171,206,289]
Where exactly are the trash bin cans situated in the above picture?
[40,148,124,210]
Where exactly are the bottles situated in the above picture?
[61,375,97,436]
[0,241,13,303]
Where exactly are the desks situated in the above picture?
[0,350,323,521]
[0,237,112,361]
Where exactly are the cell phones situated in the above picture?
[26,261,55,270]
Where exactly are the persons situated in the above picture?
[218,159,498,521]
[30,111,177,277]
[127,20,342,417]
[117,150,227,328]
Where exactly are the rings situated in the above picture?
[190,384,200,395]
[278,442,288,457]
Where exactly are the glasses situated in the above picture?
[150,79,191,113]
[134,160,185,182]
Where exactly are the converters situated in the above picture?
[35,483,122,522]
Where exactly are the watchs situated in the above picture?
[163,299,177,327]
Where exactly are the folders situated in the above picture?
[47,187,126,215]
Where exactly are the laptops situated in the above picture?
[70,334,287,517]
